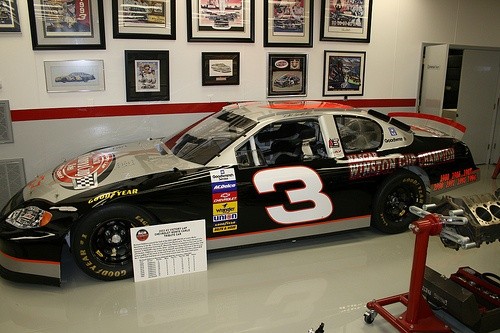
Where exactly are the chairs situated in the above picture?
[299,124,319,155]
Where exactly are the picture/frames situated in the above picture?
[202,52,240,85]
[44,59,104,93]
[27,0,106,50]
[320,0,372,43]
[264,0,313,48]
[324,49,366,96]
[0,0,22,33]
[266,51,309,98]
[186,0,255,42]
[113,0,177,41]
[125,50,169,102]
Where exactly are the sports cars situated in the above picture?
[55,71,95,83]
[0,101,484,289]
